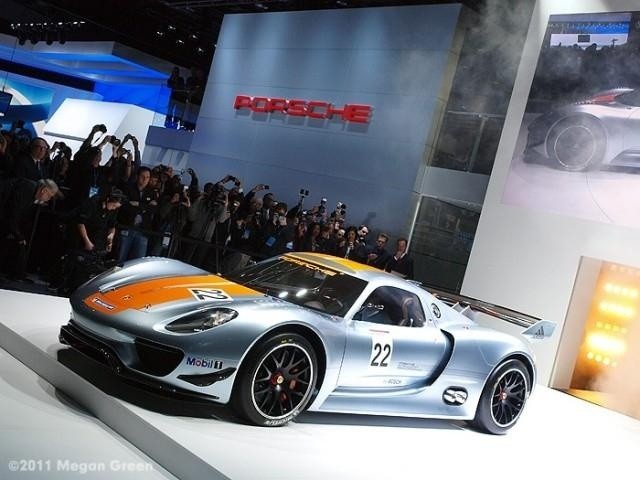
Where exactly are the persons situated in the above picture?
[183,65,206,129]
[531,41,640,111]
[164,66,186,129]
[351,291,391,323]
[1,121,413,298]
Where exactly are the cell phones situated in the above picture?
[264,185,270,190]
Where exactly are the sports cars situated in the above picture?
[522,87,640,173]
[58,251,558,435]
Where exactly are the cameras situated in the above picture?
[100,124,107,134]
[129,136,135,139]
[318,197,328,214]
[125,149,131,154]
[59,142,69,153]
[336,201,346,215]
[109,135,120,146]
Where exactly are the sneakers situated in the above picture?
[0,264,74,293]
